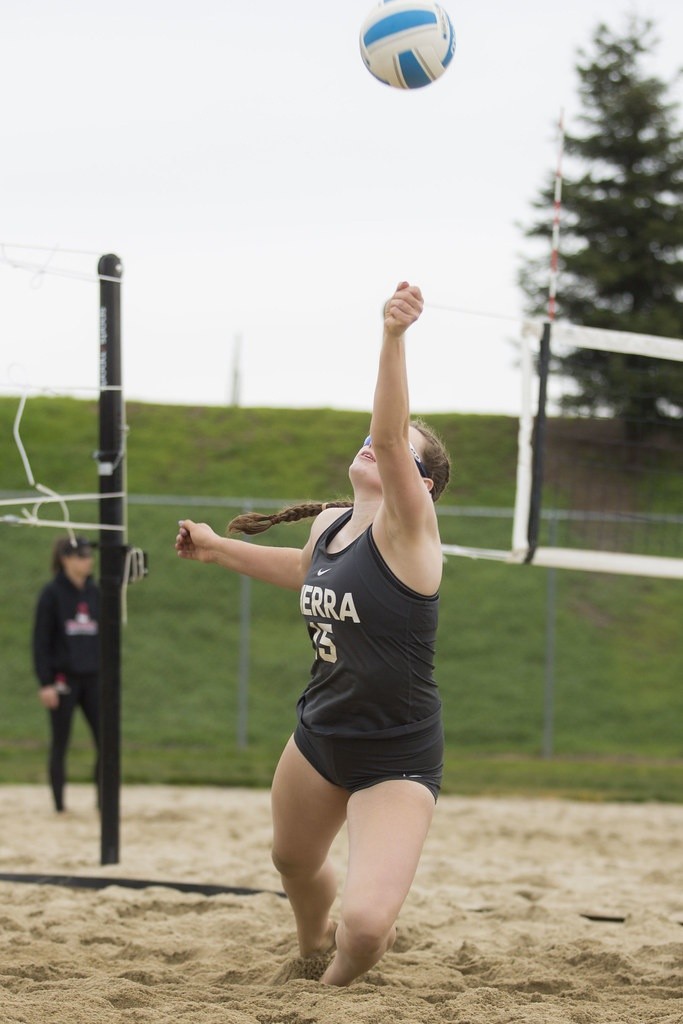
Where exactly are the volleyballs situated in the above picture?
[356,0,459,94]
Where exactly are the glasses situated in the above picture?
[364,435,427,477]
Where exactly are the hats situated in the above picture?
[58,537,100,557]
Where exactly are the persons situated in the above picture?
[32,538,105,813]
[176,276,449,992]
